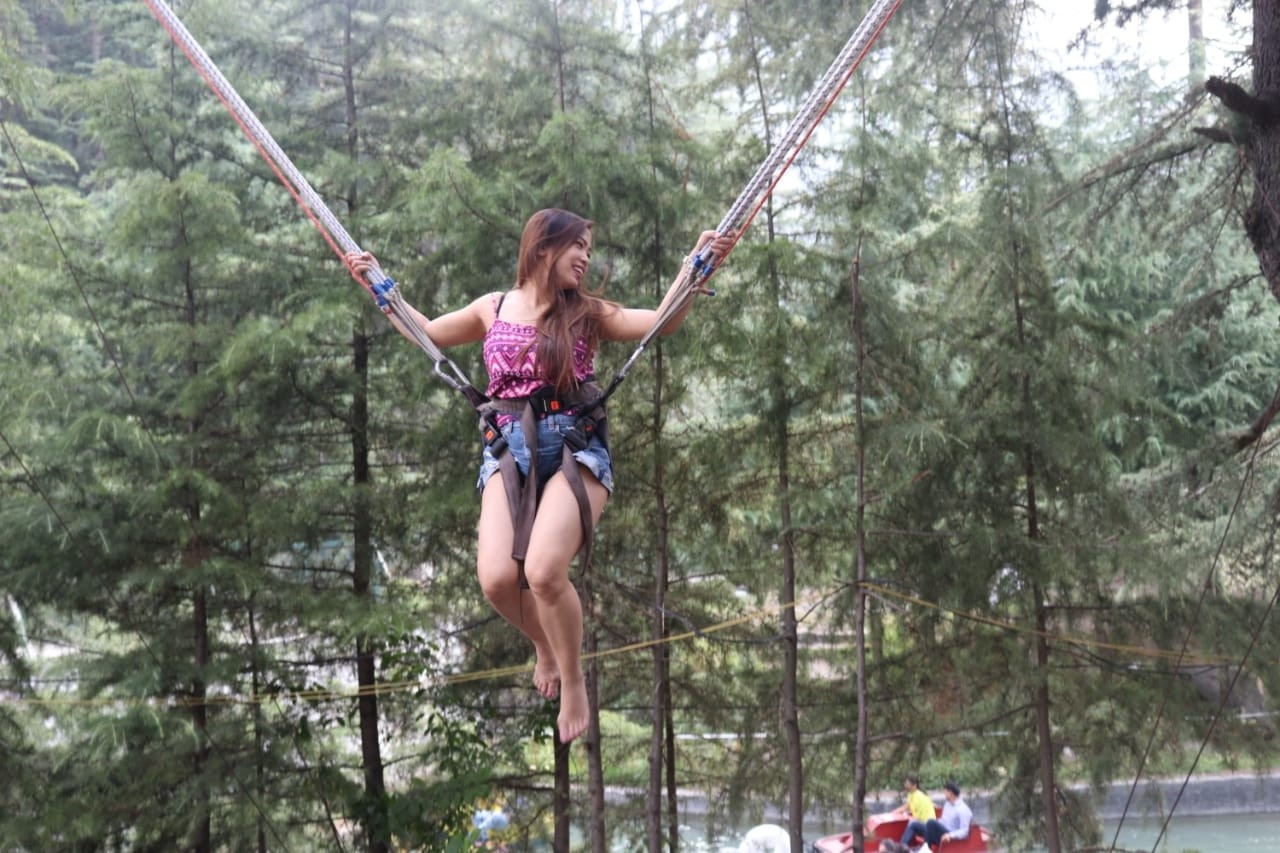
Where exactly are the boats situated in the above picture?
[812,802,1003,853]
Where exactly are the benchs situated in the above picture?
[906,808,989,853]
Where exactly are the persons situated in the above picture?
[344,208,735,744]
[893,775,936,845]
[925,782,973,851]
